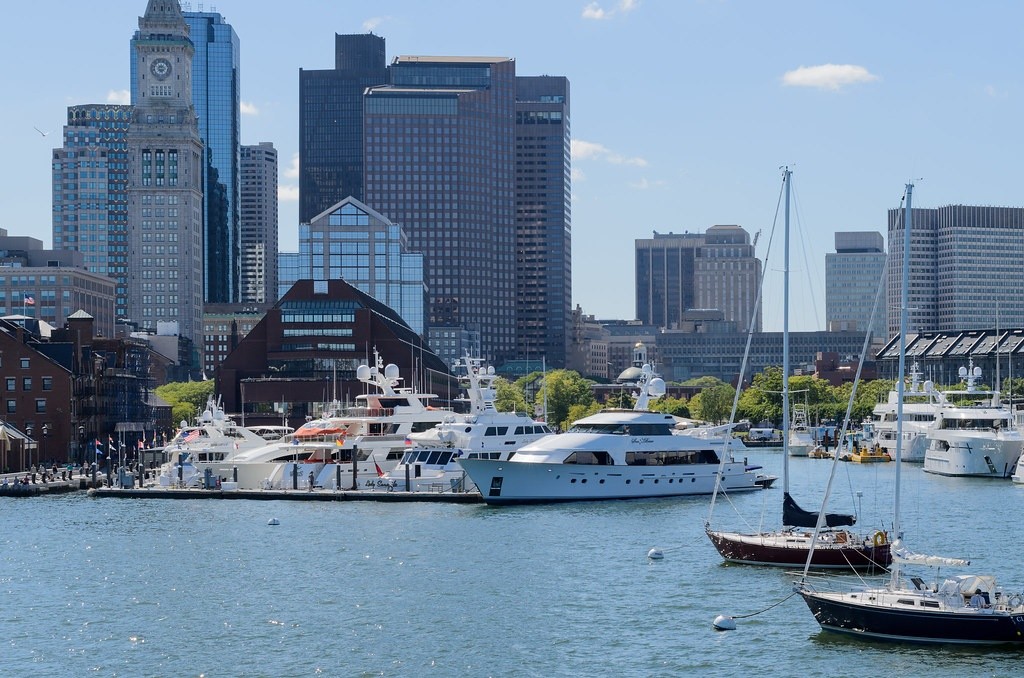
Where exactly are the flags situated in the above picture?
[181,430,199,443]
[457,449,463,455]
[404,437,412,444]
[138,441,143,448]
[294,438,299,445]
[336,439,345,446]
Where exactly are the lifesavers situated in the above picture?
[874,532,885,546]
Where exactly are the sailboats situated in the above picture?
[788,179,1024,650]
[703,163,906,571]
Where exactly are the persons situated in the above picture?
[970,589,986,609]
[3,475,29,491]
[309,471,315,492]
[30,461,100,484]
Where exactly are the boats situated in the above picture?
[157,345,778,505]
[788,349,1024,478]
